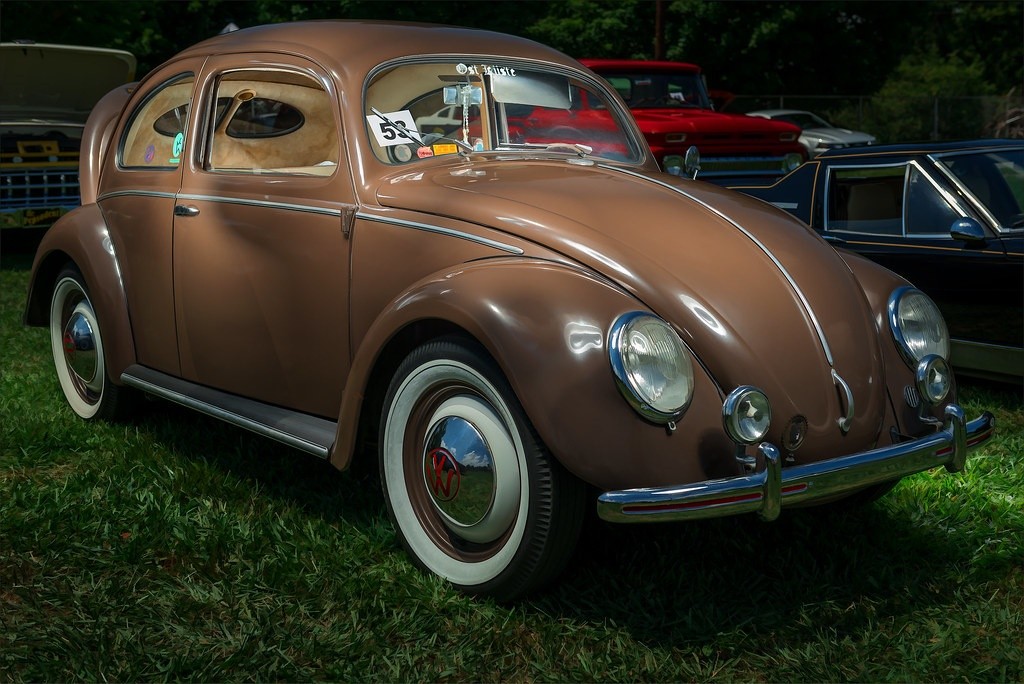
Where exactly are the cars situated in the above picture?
[743,140,1024,403]
[743,106,878,162]
[21,18,997,598]
[455,57,813,193]
[0,45,138,234]
